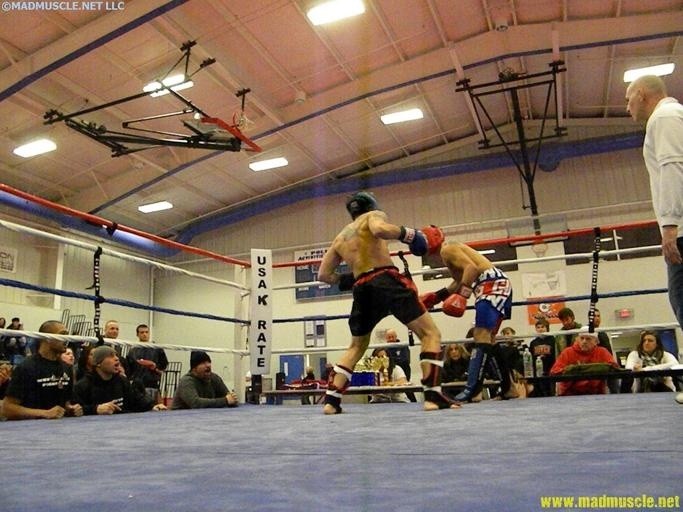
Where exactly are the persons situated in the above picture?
[419,225,520,403]
[625,74,683,403]
[0,318,238,419]
[301,363,334,405]
[371,329,410,403]
[440,308,682,398]
[317,190,461,414]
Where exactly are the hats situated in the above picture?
[191,351,211,369]
[579,326,601,345]
[92,346,118,367]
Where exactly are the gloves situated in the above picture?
[409,230,429,257]
[441,286,471,319]
[418,290,436,311]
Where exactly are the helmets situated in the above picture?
[419,225,445,256]
[347,191,377,220]
